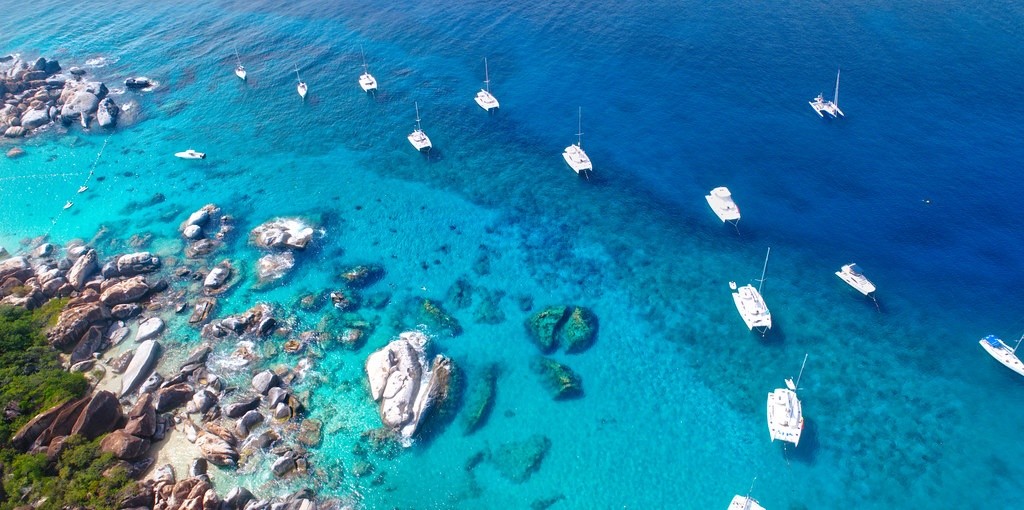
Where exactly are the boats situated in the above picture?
[704,186,742,236]
[474,57,500,112]
[809,70,845,117]
[731,247,772,338]
[834,262,877,296]
[174,149,206,160]
[233,44,247,81]
[359,43,377,93]
[407,102,433,153]
[562,106,593,180]
[766,353,809,448]
[726,493,769,510]
[292,63,308,99]
[978,333,1024,377]
